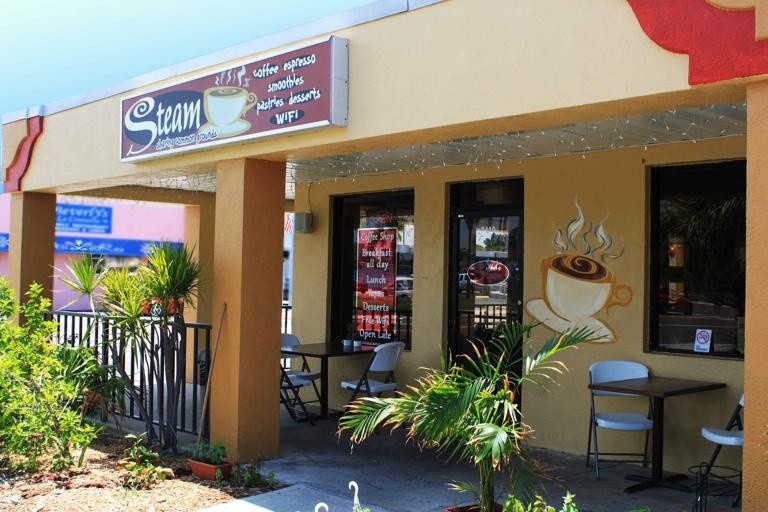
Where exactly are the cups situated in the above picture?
[344,346,361,352]
[353,340,363,347]
[545,255,635,325]
[202,87,258,127]
[340,340,352,347]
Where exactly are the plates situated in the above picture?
[522,297,619,345]
[200,121,253,140]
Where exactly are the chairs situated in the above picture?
[276,331,321,428]
[585,357,655,482]
[695,392,743,508]
[339,342,409,438]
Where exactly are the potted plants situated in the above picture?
[331,316,613,512]
[184,443,233,483]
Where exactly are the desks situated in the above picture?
[586,372,727,497]
[279,342,377,427]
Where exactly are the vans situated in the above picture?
[457,272,482,294]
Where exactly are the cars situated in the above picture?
[138,294,184,317]
[382,274,413,297]
[656,285,693,319]
